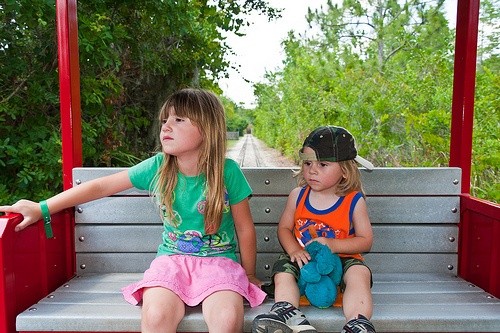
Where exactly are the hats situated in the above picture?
[299,125,374,171]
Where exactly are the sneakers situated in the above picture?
[251,301,317,333]
[339,313,375,333]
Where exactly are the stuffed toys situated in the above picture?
[298,241,342,308]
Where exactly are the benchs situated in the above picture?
[16,167,500,333]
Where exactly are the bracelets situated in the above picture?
[39,200,54,238]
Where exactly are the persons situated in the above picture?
[252,126,376,333]
[1,88,267,333]
